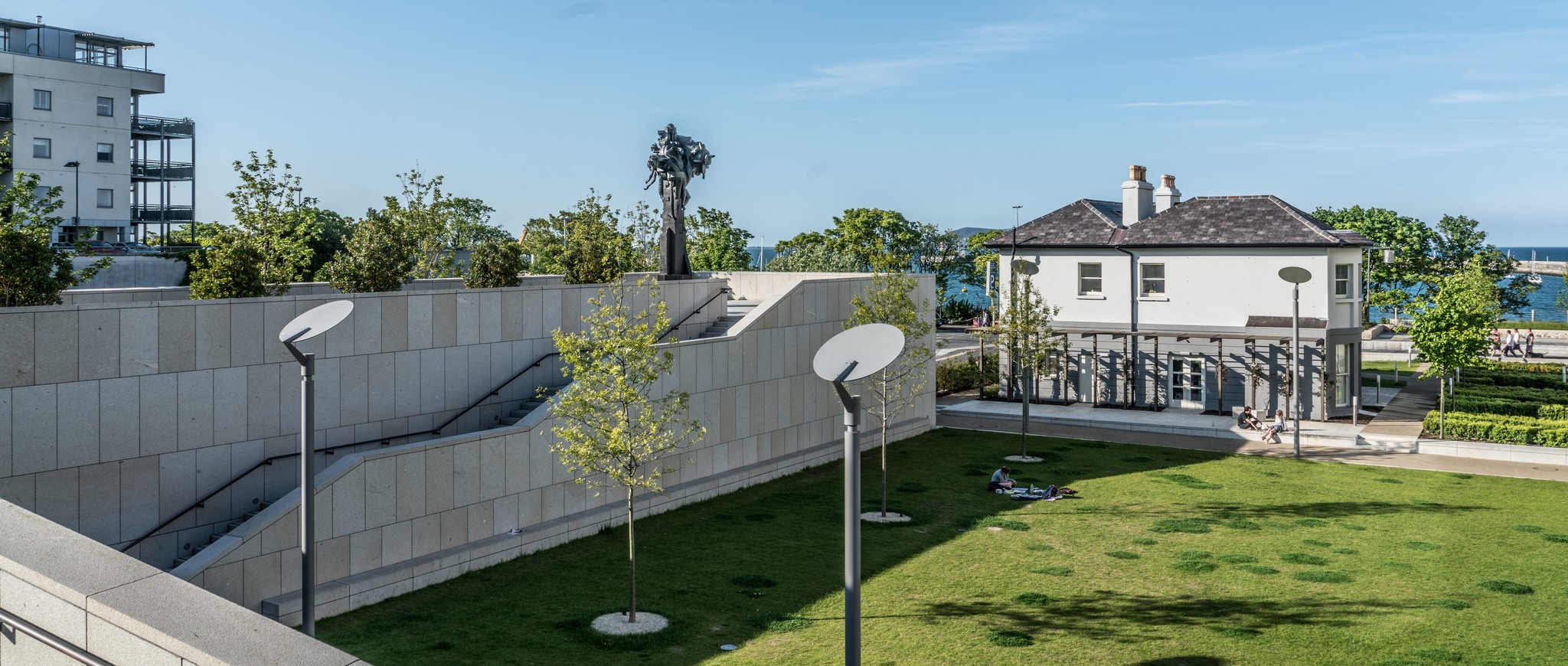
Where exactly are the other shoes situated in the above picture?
[1247,426,1252,430]
[1262,436,1264,440]
[1265,438,1267,444]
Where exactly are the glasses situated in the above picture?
[1276,413,1278,415]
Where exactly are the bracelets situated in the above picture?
[1260,422,1261,423]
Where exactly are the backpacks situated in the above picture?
[1057,488,1073,495]
[1041,484,1058,499]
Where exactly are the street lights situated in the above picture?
[1011,259,1040,459]
[1278,267,1313,457]
[63,160,80,241]
[278,298,357,639]
[811,323,908,666]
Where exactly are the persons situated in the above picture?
[1490,329,1504,357]
[644,144,691,210]
[1511,328,1525,358]
[983,311,991,333]
[1502,330,1518,357]
[1237,406,1262,432]
[991,466,1016,488]
[982,310,988,333]
[1522,328,1534,355]
[1262,410,1292,444]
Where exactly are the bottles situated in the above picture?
[1030,484,1034,493]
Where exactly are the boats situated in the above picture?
[1504,274,1516,280]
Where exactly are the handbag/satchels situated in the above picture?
[996,489,1003,494]
[988,481,1004,492]
[1261,423,1269,431]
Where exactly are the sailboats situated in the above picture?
[961,272,969,292]
[1527,249,1543,284]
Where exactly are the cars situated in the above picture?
[48,241,223,254]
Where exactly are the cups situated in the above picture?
[1057,495,1063,499]
[1050,497,1058,500]
